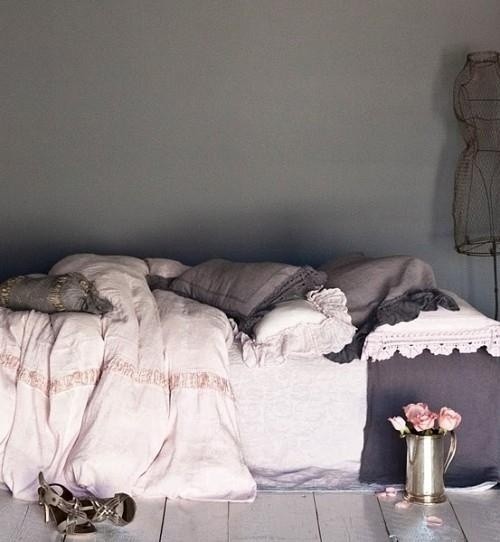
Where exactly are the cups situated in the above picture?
[402,429,457,504]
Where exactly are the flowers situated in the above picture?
[387,401,463,436]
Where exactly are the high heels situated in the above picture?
[37,472,97,536]
[79,492,136,527]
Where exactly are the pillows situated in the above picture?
[0,272,114,316]
